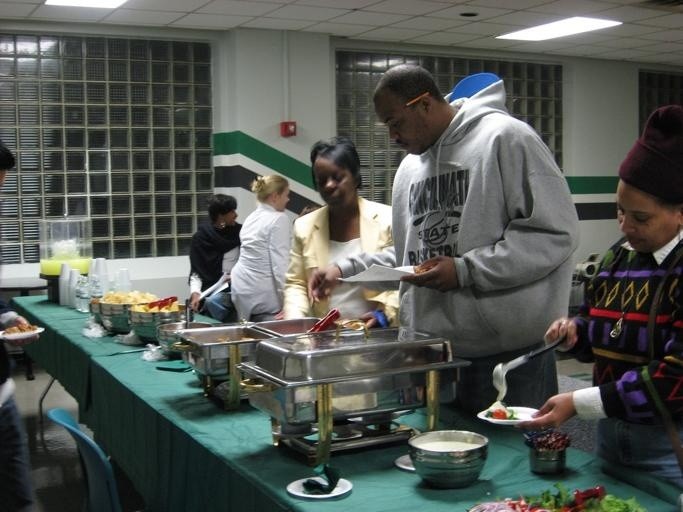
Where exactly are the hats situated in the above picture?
[450,73,500,101]
[619,106,683,203]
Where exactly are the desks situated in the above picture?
[0,278,49,380]
[10,295,683,512]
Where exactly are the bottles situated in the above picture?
[73,274,104,313]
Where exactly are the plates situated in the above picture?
[284,476,353,500]
[334,318,363,330]
[0,328,45,340]
[393,455,415,473]
[476,406,540,426]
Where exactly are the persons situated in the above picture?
[187,194,240,314]
[230,175,296,321]
[517,105,683,491]
[1,146,39,511]
[282,136,398,329]
[299,206,323,215]
[310,63,582,413]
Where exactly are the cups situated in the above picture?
[523,434,570,476]
[86,256,134,294]
[57,262,81,307]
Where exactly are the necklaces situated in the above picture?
[609,248,662,338]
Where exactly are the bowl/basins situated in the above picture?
[406,429,490,490]
[155,321,214,358]
[85,297,149,333]
[127,302,188,344]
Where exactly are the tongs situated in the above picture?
[305,308,340,333]
